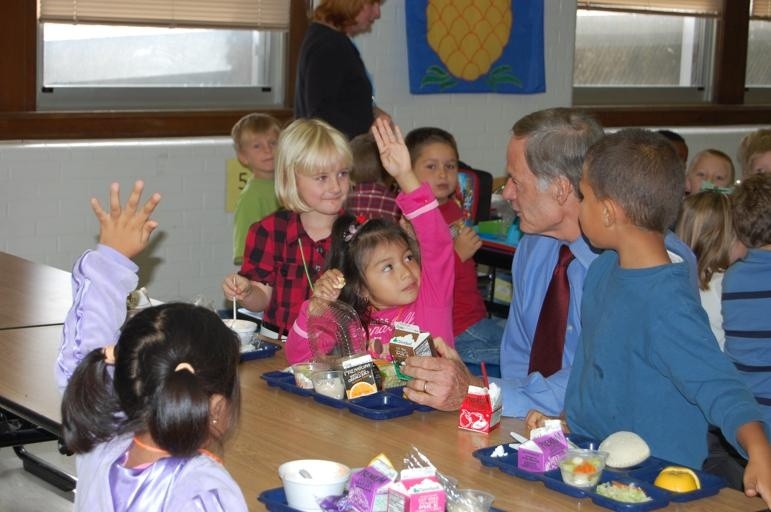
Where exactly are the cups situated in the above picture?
[560,449,611,462]
[289,362,327,388]
[446,488,497,512]
[557,455,605,488]
[311,370,348,400]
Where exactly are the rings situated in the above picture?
[423,381,428,393]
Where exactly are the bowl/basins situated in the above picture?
[220,317,258,345]
[277,458,350,510]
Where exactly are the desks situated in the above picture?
[474,218,517,320]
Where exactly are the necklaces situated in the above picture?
[126,428,225,466]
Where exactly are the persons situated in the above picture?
[672,187,747,350]
[527,130,771,512]
[57,178,251,512]
[221,117,353,333]
[227,107,288,265]
[399,126,508,367]
[685,147,733,193]
[661,128,689,171]
[293,0,393,139]
[282,117,458,363]
[736,129,771,179]
[341,130,403,227]
[721,173,771,442]
[400,108,607,422]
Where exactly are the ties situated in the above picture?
[528,244,576,377]
